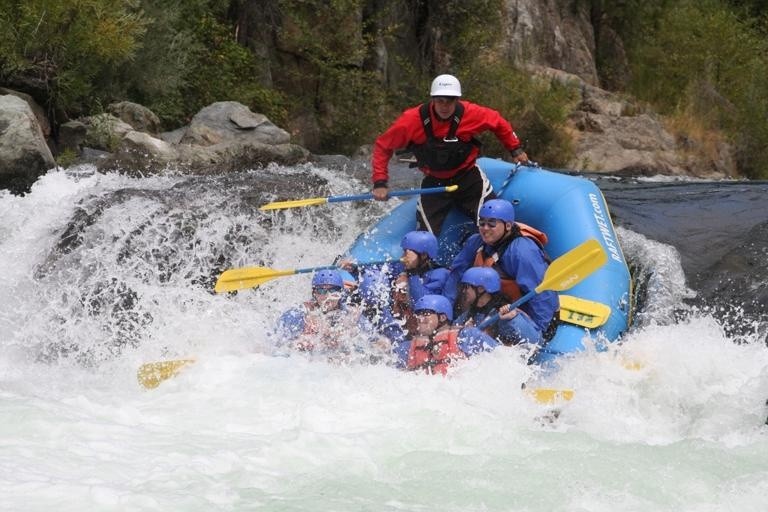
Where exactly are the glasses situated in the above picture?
[479,218,504,227]
[314,287,341,293]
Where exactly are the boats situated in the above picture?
[272,155,637,384]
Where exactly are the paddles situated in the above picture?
[138,361,195,389]
[215,258,404,292]
[258,185,459,211]
[558,294,610,328]
[476,240,607,328]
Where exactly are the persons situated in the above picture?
[452,267,547,346]
[389,229,454,333]
[392,294,501,376]
[450,199,560,343]
[372,74,529,231]
[273,272,392,366]
[342,256,402,341]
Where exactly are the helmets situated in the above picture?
[413,293,454,321]
[429,73,463,99]
[313,268,344,293]
[358,268,392,305]
[398,230,440,261]
[479,198,515,226]
[460,265,502,295]
